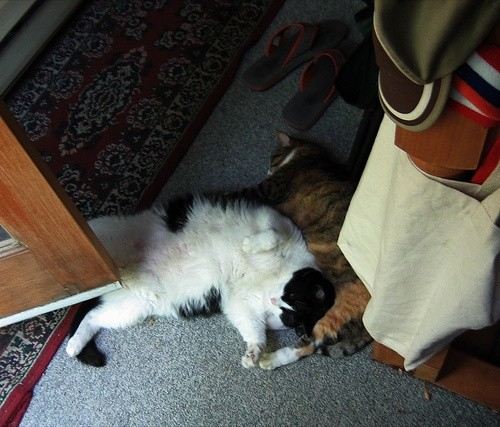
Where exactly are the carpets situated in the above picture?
[0,0,288,427]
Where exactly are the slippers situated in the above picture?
[244,23,322,92]
[282,35,356,130]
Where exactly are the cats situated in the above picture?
[52,130,371,369]
